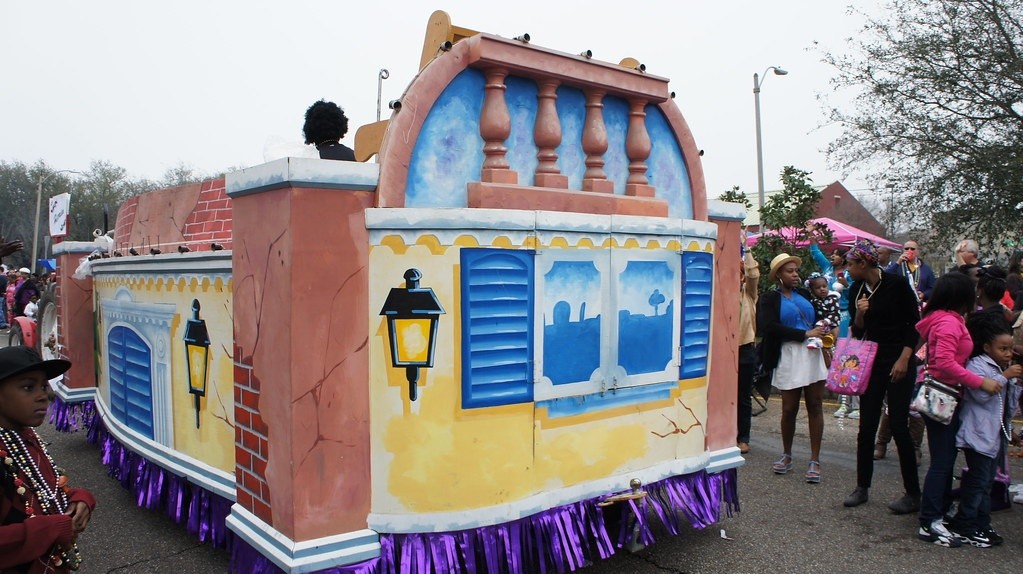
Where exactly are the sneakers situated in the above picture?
[848,410,860,419]
[833,404,849,418]
[949,525,1003,548]
[844,487,868,506]
[943,507,958,524]
[890,492,921,514]
[919,519,961,548]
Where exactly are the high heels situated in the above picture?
[804,461,821,482]
[773,453,793,473]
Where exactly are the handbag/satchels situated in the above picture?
[824,326,878,396]
[909,378,961,425]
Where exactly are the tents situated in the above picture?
[744,216,905,262]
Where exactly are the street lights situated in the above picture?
[754,64,790,233]
[31,168,81,275]
[885,183,896,242]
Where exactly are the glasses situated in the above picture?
[904,247,915,251]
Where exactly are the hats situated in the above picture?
[0,345,72,379]
[847,238,879,264]
[767,253,802,281]
[19,267,30,274]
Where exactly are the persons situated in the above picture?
[303,101,356,163]
[0,345,97,574]
[756,252,839,483]
[809,232,1023,419]
[841,239,923,514]
[737,224,760,452]
[0,266,51,334]
[916,271,1003,548]
[955,320,1023,547]
[802,274,840,349]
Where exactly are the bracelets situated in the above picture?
[744,248,751,255]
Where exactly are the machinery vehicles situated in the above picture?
[8,210,110,400]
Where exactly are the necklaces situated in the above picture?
[0,426,85,573]
[992,365,1013,442]
[318,139,340,147]
[855,268,881,308]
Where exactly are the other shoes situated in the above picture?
[874,445,887,459]
[916,450,921,466]
[738,441,749,453]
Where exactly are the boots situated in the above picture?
[990,480,1012,513]
[953,468,970,501]
